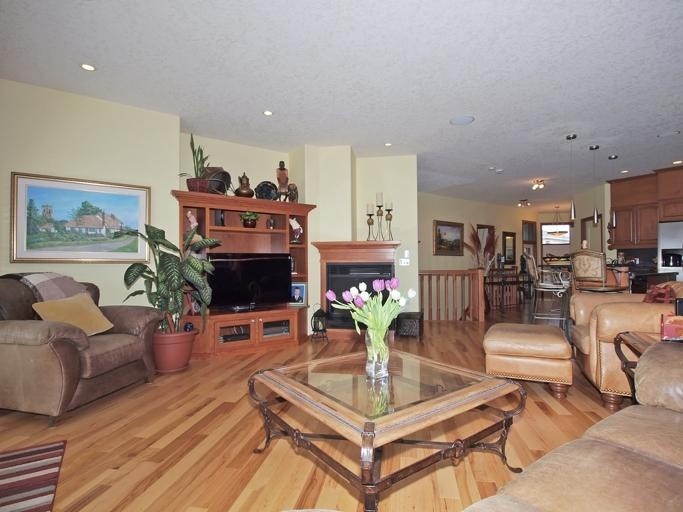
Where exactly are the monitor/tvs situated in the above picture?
[207,253,291,312]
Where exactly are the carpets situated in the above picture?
[1,439,66,512]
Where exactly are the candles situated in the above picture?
[385,200,391,209]
[366,203,373,214]
[374,192,383,206]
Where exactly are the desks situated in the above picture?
[608,264,678,293]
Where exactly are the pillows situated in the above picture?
[30,293,113,337]
[641,286,676,303]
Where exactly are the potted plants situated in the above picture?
[239,211,259,228]
[177,132,208,192]
[119,225,221,375]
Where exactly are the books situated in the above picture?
[660,314,682,341]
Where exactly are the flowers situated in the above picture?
[324,277,417,362]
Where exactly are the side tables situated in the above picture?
[614,331,661,406]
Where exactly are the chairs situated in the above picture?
[523,254,568,324]
[569,250,628,292]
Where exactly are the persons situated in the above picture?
[290,285,305,301]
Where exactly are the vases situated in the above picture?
[364,329,390,379]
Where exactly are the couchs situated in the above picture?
[465,341,681,511]
[483,323,572,400]
[568,280,681,406]
[0,273,162,429]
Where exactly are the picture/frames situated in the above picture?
[432,220,464,257]
[502,231,515,264]
[10,170,152,263]
[476,225,495,263]
[287,282,307,306]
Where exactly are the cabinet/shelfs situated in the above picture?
[170,189,316,356]
[481,265,528,309]
[605,174,656,250]
[311,241,402,329]
[654,165,683,222]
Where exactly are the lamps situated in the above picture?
[588,145,599,223]
[565,134,576,220]
[530,179,543,190]
[607,153,618,227]
[545,203,568,238]
[517,198,530,207]
